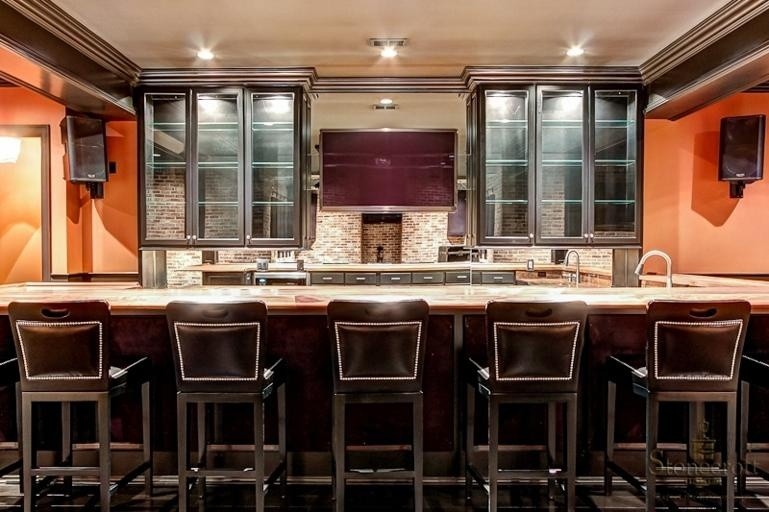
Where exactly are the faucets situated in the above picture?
[634,250,673,288]
[564,249,581,284]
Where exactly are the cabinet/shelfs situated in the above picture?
[202,272,252,286]
[312,271,515,285]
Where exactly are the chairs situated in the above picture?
[457,300,590,512]
[605,299,750,512]
[7,301,152,512]
[166,300,289,512]
[0,358,22,493]
[327,299,427,512]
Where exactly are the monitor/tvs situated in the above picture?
[317,127,460,214]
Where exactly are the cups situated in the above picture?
[297,259,305,272]
[256,257,269,271]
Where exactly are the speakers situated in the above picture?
[718,114,766,198]
[58,116,111,200]
